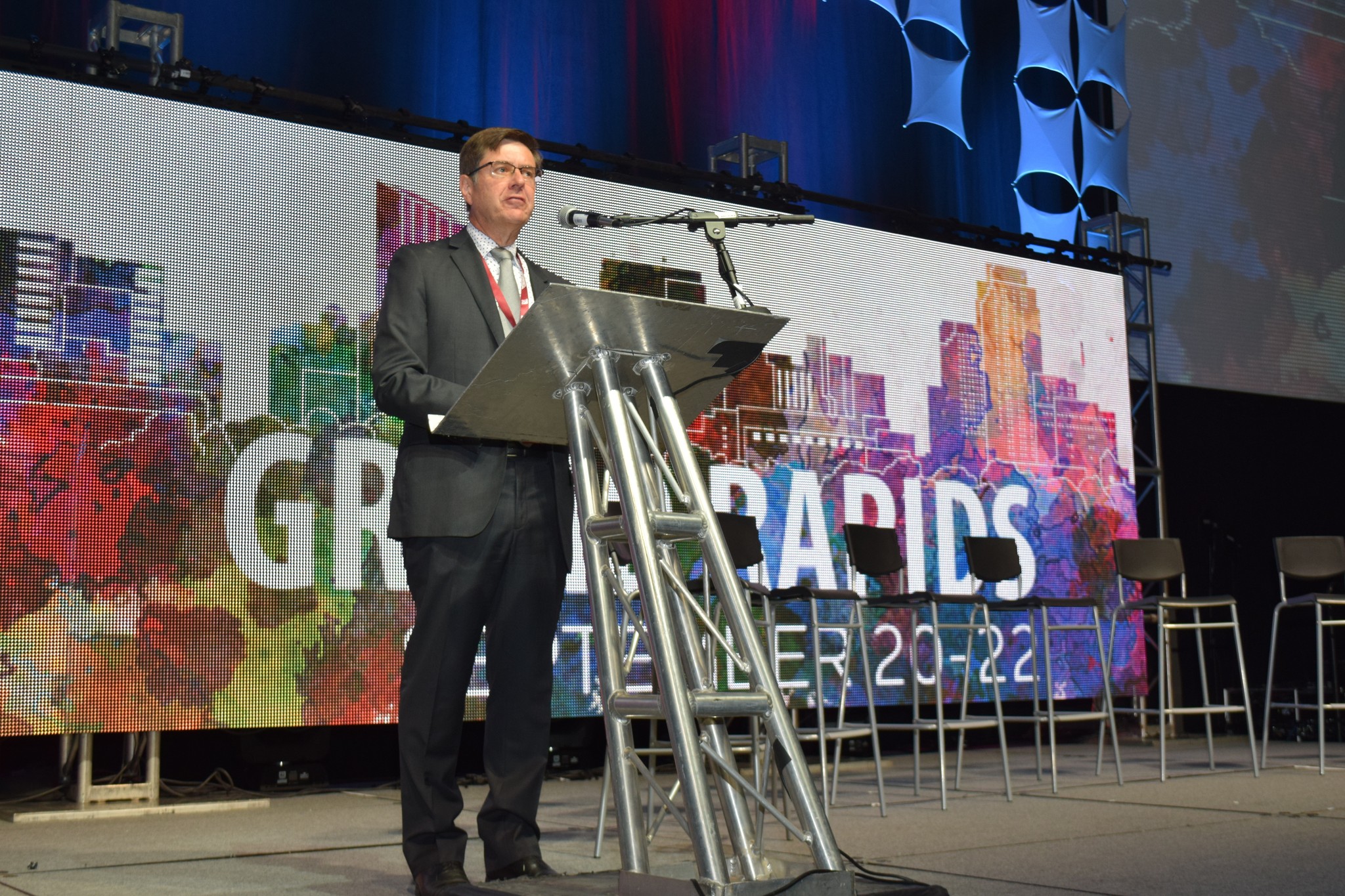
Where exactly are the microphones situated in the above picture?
[558,204,620,229]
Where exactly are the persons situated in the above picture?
[372,128,576,896]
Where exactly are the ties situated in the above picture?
[490,247,521,338]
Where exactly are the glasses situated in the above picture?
[466,160,544,183]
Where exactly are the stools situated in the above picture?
[1096,537,1258,781]
[1256,534,1344,776]
[705,512,893,814]
[591,502,758,858]
[952,538,1121,802]
[833,510,1016,810]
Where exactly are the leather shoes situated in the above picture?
[414,870,468,896]
[484,856,559,884]
